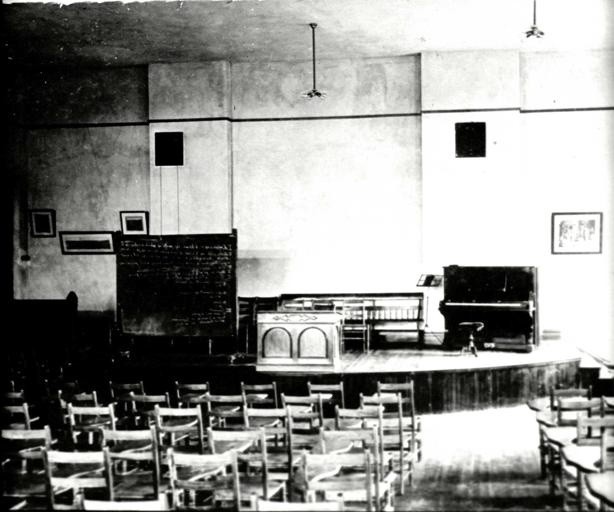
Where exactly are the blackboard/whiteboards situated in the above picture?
[115,234,238,336]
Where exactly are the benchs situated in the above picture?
[280,291,426,356]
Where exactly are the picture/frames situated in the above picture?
[551,212,603,254]
[28,209,149,257]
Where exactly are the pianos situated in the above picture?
[438,265,538,353]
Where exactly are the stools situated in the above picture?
[457,321,484,356]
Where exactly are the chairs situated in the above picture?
[527,384,614,512]
[237,297,277,353]
[1,359,424,512]
[282,300,370,353]
